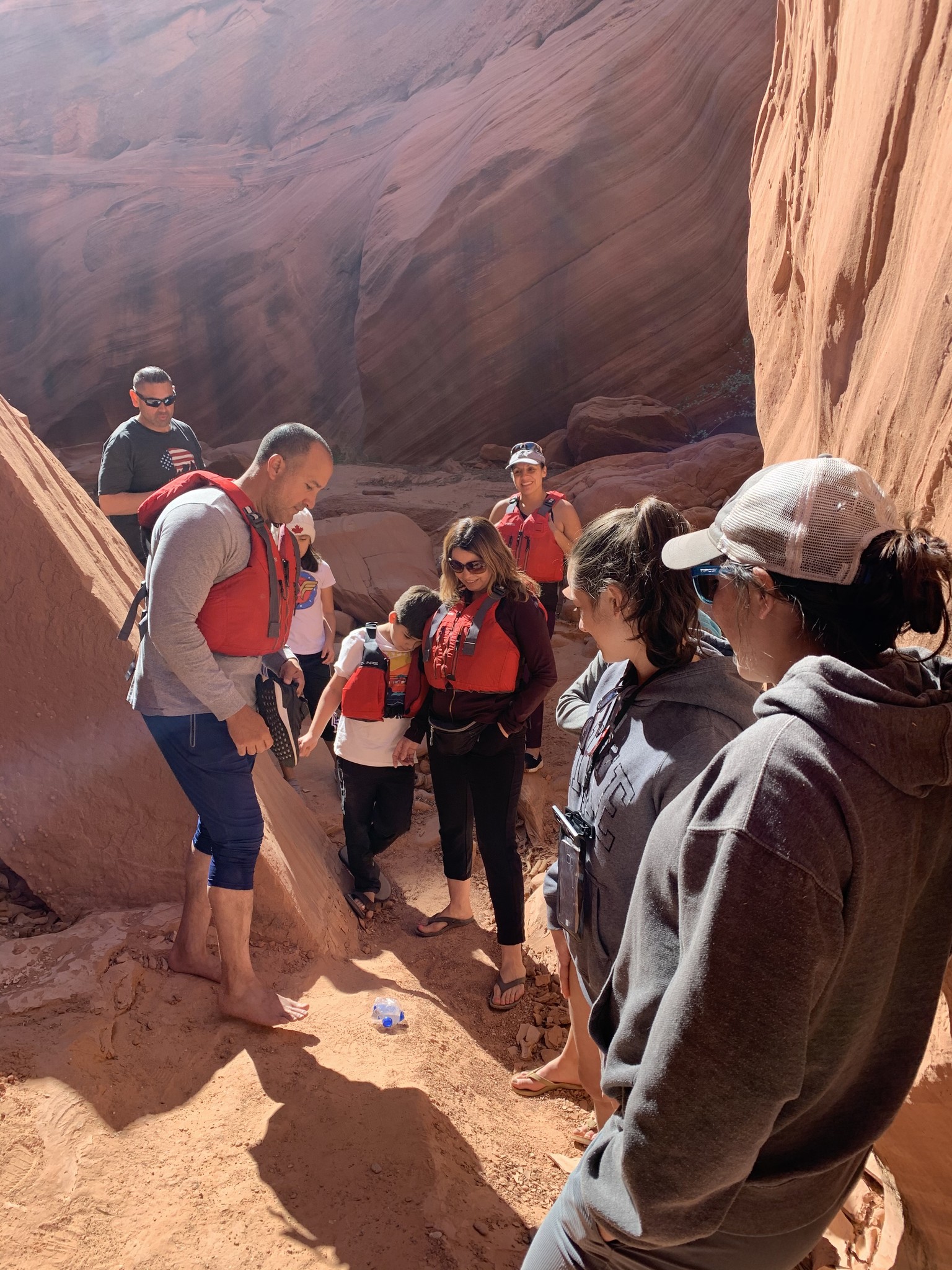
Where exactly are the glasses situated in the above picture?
[577,685,628,756]
[447,558,487,574]
[690,563,784,604]
[510,443,543,456]
[133,386,177,407]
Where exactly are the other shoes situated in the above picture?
[333,764,341,800]
[283,776,307,806]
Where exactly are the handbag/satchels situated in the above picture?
[426,716,532,757]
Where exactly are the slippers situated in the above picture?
[509,1064,585,1096]
[415,912,474,937]
[489,972,526,1010]
[571,1120,598,1146]
[344,891,377,919]
[338,846,392,902]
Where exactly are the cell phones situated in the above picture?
[556,839,584,934]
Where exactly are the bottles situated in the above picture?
[372,996,403,1027]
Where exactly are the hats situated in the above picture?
[562,586,573,601]
[286,507,316,545]
[661,453,907,585]
[505,441,546,471]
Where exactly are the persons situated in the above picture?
[519,453,952,1268]
[392,516,559,1011]
[97,365,206,567]
[510,493,767,1145]
[286,507,336,759]
[126,422,335,1028]
[297,585,443,920]
[489,441,584,772]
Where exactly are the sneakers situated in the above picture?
[255,673,310,768]
[523,751,544,773]
